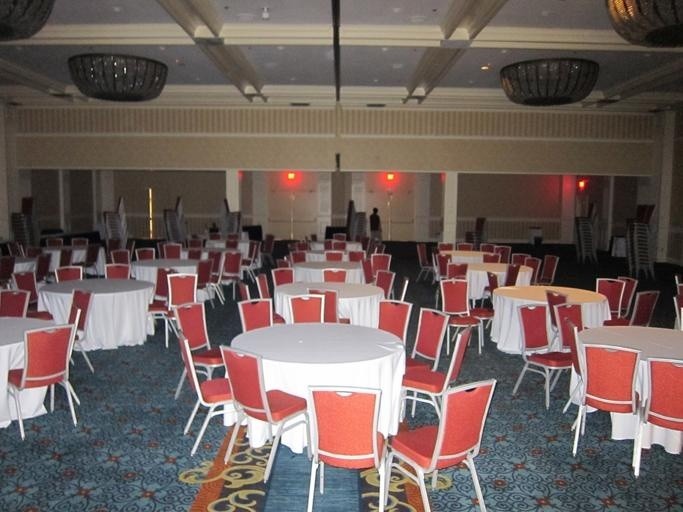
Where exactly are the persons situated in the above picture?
[210,222,218,232]
[370,209,383,249]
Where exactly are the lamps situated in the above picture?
[1,1,57,41]
[66,52,168,106]
[501,55,599,110]
[601,0,682,52]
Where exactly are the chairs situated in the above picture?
[0,192,680,511]
